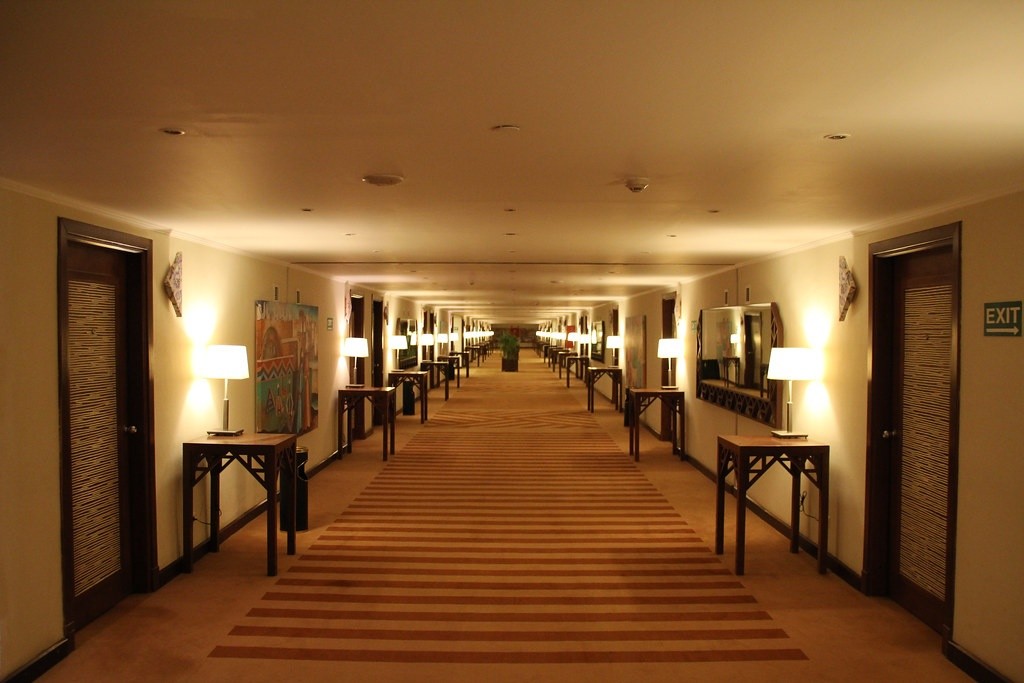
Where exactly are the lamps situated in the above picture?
[438,330,494,355]
[656,337,680,389]
[605,336,621,368]
[421,334,435,362]
[342,338,369,388]
[536,330,592,356]
[766,347,814,439]
[391,335,408,372]
[202,344,250,436]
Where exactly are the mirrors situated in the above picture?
[695,302,782,429]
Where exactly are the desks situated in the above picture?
[181,433,296,576]
[630,388,684,462]
[388,371,428,424]
[587,366,623,414]
[420,362,450,401]
[437,340,494,389]
[714,434,830,576]
[723,356,740,388]
[532,340,589,388]
[337,387,396,461]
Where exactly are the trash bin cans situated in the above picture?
[624,389,635,427]
[446,364,454,380]
[280,446,309,532]
[403,381,414,415]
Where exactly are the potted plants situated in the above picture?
[499,335,520,373]
